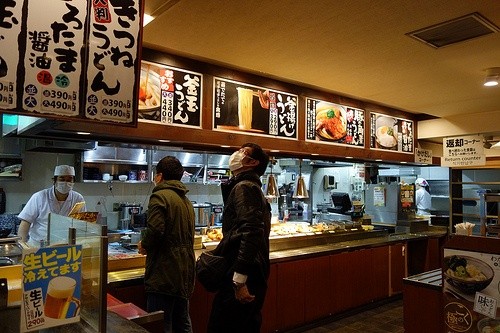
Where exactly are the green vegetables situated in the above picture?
[446,255,467,271]
[388,126,394,136]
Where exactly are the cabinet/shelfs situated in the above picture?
[75,142,233,186]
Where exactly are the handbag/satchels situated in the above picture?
[196,251,224,292]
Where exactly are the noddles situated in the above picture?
[446,264,489,280]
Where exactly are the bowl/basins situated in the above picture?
[138,67,162,121]
[316,107,348,142]
[375,139,398,149]
[102,172,113,181]
[217,125,264,133]
[0,224,13,237]
[119,175,128,181]
[443,255,494,295]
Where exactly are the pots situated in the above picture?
[189,201,223,227]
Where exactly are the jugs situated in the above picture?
[107,211,118,230]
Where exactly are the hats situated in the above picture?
[53,165,75,176]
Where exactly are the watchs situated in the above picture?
[232,281,246,291]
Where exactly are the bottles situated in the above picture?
[280,200,290,221]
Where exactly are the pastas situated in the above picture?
[237,87,254,129]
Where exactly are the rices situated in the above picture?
[377,128,397,146]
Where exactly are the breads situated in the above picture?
[316,102,340,120]
[207,228,224,240]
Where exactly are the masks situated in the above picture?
[54,181,75,194]
[228,150,254,171]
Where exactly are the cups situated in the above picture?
[44,276,80,319]
[125,232,142,243]
[119,237,131,246]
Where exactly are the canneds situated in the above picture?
[129,169,146,181]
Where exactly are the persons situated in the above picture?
[16,164,88,243]
[207,142,272,333]
[414,177,432,224]
[136,155,198,333]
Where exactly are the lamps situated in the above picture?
[292,159,310,199]
[265,156,281,198]
[484,75,500,86]
